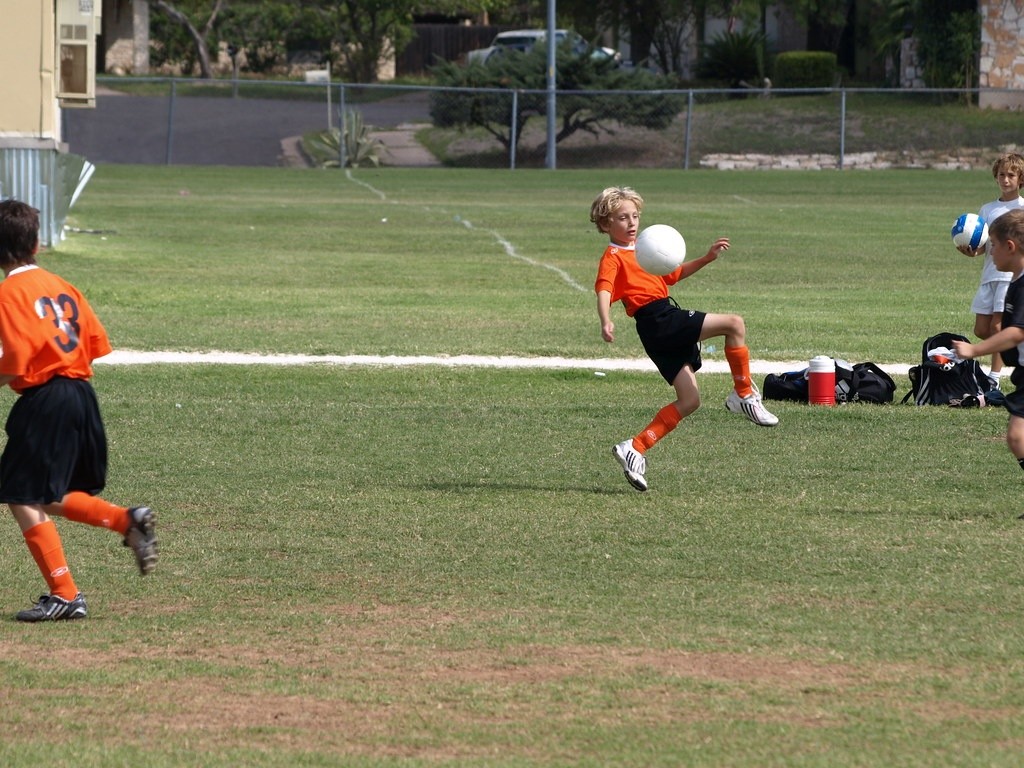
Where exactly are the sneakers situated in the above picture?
[123,507,159,575]
[611,439,648,491]
[16,592,88,621]
[726,377,779,426]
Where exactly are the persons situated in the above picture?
[0,198,158,622]
[951,208,1024,472]
[589,186,779,491]
[957,152,1024,389]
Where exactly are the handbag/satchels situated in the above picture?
[762,357,897,405]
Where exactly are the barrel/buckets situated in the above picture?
[804,355,835,406]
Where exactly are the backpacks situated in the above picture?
[909,332,991,406]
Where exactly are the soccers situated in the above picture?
[950,212,989,251]
[635,224,687,276]
